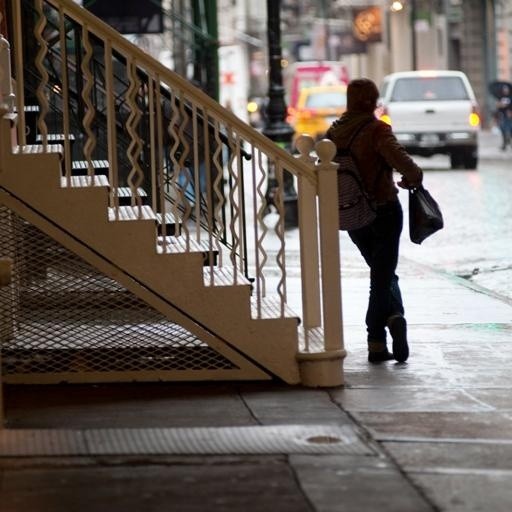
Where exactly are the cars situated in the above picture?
[247,82,270,129]
[289,84,351,154]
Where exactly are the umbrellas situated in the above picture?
[487,81,512,103]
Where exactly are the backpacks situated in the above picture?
[319,119,386,230]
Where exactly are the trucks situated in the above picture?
[282,59,351,124]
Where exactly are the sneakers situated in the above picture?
[368,314,408,362]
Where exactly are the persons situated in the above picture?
[325,77,424,363]
[164,79,208,218]
[496,85,512,150]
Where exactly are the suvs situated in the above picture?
[373,69,482,172]
